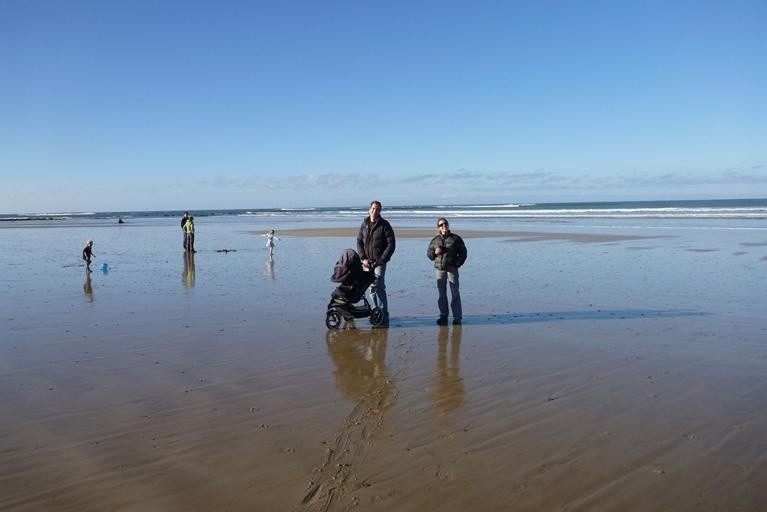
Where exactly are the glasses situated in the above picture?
[437,221,449,228]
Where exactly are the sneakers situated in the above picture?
[435,317,463,327]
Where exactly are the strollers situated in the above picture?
[325,248,384,329]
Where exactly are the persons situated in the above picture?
[356,201,396,324]
[424,324,466,416]
[426,217,467,325]
[180,211,189,250]
[182,216,196,253]
[83,270,95,304]
[364,325,398,412]
[264,256,276,279]
[181,250,188,287]
[80,238,97,272]
[186,252,195,288]
[258,229,280,256]
